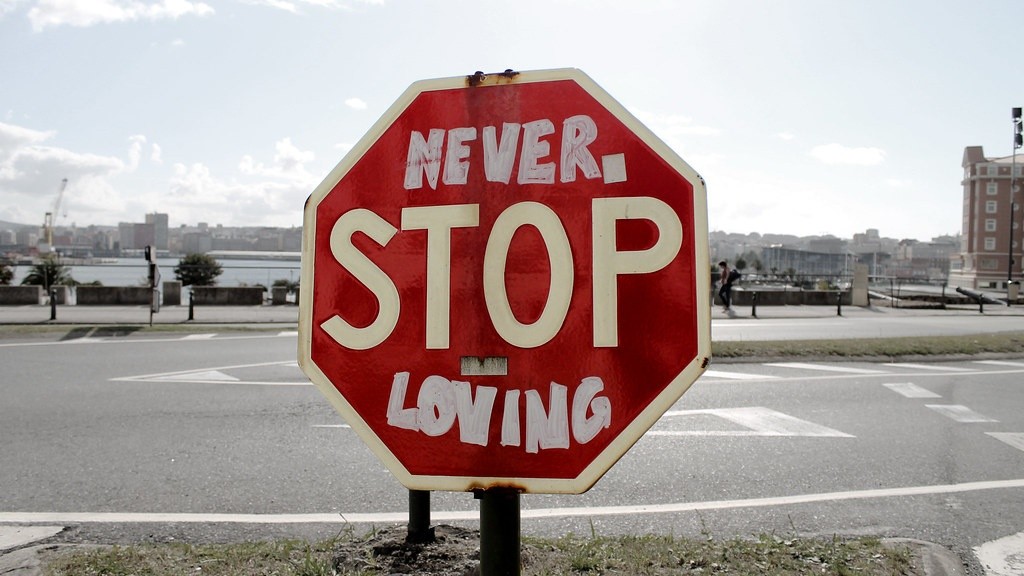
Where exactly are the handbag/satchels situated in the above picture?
[727,269,740,283]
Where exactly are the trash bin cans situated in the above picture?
[1007,280,1020,301]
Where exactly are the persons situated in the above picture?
[711,261,736,314]
